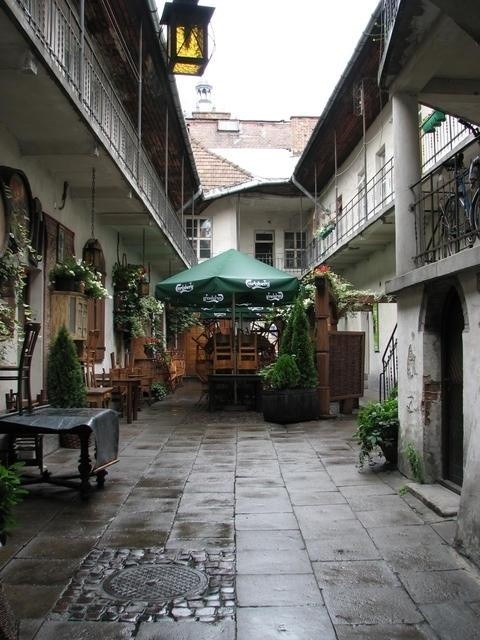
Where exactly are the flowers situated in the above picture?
[49,255,114,302]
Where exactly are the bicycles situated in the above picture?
[442,118,480,254]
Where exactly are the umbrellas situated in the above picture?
[155,248,300,404]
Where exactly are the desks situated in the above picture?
[0,406,119,500]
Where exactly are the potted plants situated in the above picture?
[260,299,317,424]
[46,324,92,449]
[351,394,397,473]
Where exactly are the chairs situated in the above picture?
[237,328,258,373]
[79,351,153,425]
[166,352,185,394]
[0,322,41,415]
[75,329,100,390]
[212,326,234,373]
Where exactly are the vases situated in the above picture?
[54,280,85,294]
